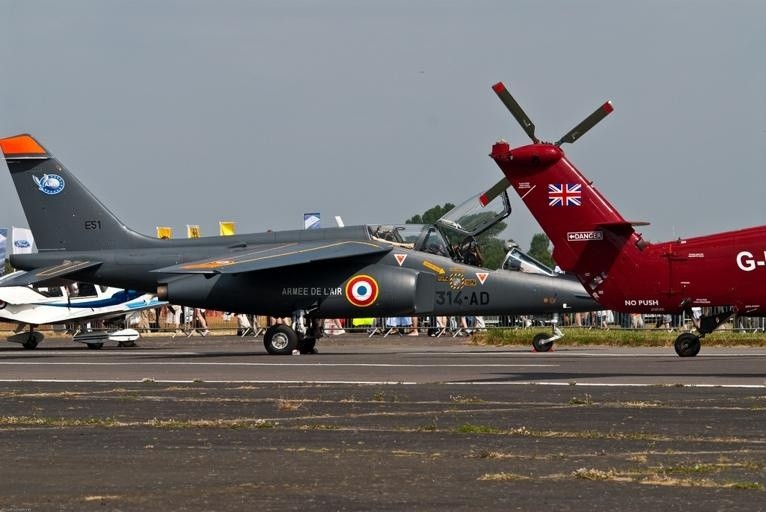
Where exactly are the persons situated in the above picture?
[332,316,485,337]
[136,305,209,337]
[236,313,293,336]
[690,307,701,333]
[519,309,672,333]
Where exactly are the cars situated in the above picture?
[479,309,763,334]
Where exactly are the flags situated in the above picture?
[11,226,34,254]
[155,226,170,239]
[302,213,321,229]
[187,225,199,238]
[220,222,234,236]
[0,228,7,277]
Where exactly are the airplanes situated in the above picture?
[0,135,610,357]
[0,223,170,349]
[475,78,766,360]
